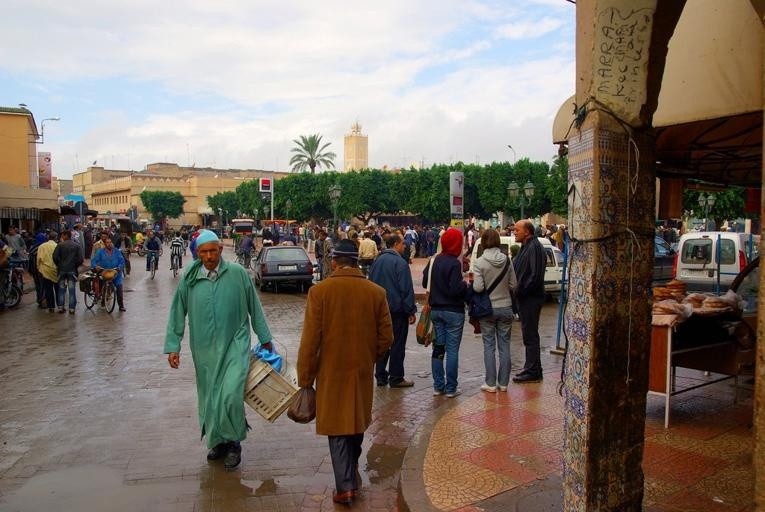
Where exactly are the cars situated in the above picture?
[252,246,313,292]
[219,224,233,238]
[653,236,677,280]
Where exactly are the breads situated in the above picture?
[652,279,728,315]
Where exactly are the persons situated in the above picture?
[371,231,417,388]
[466,229,518,394]
[654,223,679,248]
[421,228,472,399]
[163,229,272,467]
[2,216,204,314]
[466,222,569,253]
[315,223,439,281]
[223,218,319,268]
[296,238,395,505]
[505,218,547,385]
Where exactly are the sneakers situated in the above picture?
[58,309,66,313]
[515,368,542,379]
[496,382,507,392]
[446,389,461,397]
[433,388,446,396]
[512,374,540,383]
[480,383,496,392]
[390,377,415,387]
[69,308,75,314]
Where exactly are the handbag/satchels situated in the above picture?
[415,303,433,347]
[465,283,492,320]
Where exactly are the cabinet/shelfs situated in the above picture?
[647,302,742,427]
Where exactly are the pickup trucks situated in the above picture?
[176,224,222,240]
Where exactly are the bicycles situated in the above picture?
[234,248,259,269]
[140,248,163,280]
[83,266,119,313]
[116,248,132,276]
[170,247,186,277]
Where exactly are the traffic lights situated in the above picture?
[260,179,271,191]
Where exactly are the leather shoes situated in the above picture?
[119,307,126,311]
[333,489,355,503]
[207,443,226,460]
[224,445,242,468]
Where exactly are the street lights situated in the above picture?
[252,208,258,220]
[236,210,241,218]
[698,194,717,231]
[218,207,223,225]
[262,206,270,220]
[327,184,342,226]
[284,199,291,228]
[505,180,536,220]
[507,145,515,164]
[214,174,219,192]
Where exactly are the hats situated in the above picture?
[196,229,219,248]
[102,269,118,281]
[327,239,360,260]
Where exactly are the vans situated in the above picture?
[674,232,758,284]
[469,237,569,301]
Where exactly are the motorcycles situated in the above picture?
[0,257,23,308]
[136,242,163,256]
